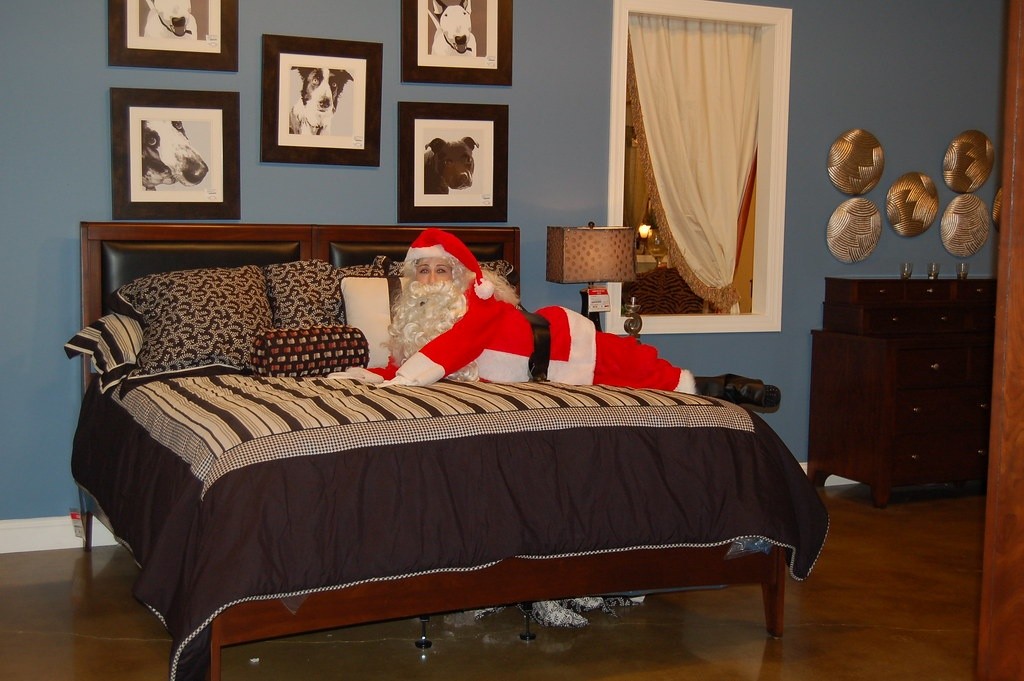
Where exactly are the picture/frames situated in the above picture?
[108,0,239,73]
[401,0,514,86]
[109,87,241,220]
[260,33,384,166]
[397,100,510,223]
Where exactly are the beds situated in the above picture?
[65,220,829,680]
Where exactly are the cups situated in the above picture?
[900,262,913,279]
[927,263,940,279]
[956,262,970,279]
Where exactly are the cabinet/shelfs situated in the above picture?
[806,278,996,507]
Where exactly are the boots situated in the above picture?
[693,373,781,413]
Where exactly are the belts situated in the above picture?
[517,308,550,382]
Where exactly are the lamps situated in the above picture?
[545,224,639,331]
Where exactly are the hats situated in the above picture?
[404,227,495,299]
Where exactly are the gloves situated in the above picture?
[375,374,420,388]
[322,375,360,380]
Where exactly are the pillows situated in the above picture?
[63,258,520,402]
[622,267,705,315]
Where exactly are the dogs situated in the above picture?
[290,65,354,135]
[141,121,209,193]
[425,137,479,193]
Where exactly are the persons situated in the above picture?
[327,227,782,415]
